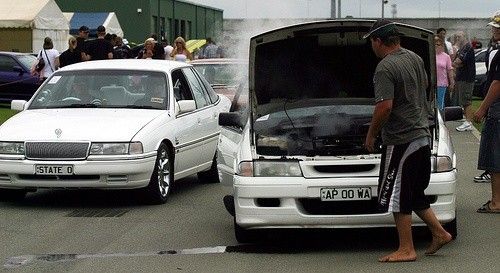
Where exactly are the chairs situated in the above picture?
[100,84,131,105]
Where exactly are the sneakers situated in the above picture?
[455,120,473,131]
[473,170,491,183]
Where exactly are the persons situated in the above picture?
[73,76,103,105]
[433,11,500,213]
[86,25,113,60]
[363,18,452,262]
[76,26,91,61]
[134,74,166,108]
[30,37,60,84]
[59,37,81,68]
[104,34,237,59]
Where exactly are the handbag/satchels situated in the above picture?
[36,50,45,72]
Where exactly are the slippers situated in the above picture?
[477,200,500,213]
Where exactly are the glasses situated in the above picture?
[176,42,182,44]
[434,44,440,46]
[441,33,445,35]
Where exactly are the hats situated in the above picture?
[80,26,90,33]
[44,37,51,43]
[161,39,168,45]
[97,26,105,32]
[104,33,117,41]
[362,20,400,39]
[122,39,128,44]
[434,36,442,41]
[151,34,158,41]
[485,10,500,28]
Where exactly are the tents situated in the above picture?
[63,12,125,38]
[0,0,71,53]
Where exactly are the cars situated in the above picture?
[174,58,249,111]
[216,18,463,246]
[473,48,488,100]
[0,59,232,205]
[0,51,48,104]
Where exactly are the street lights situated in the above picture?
[382,0,389,19]
